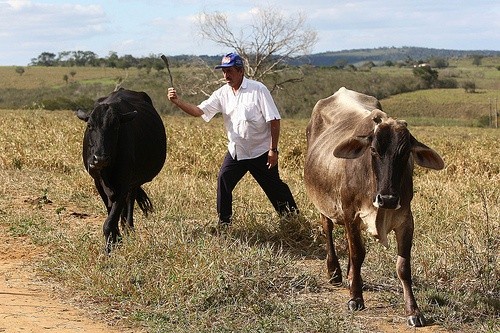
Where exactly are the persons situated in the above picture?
[167,52,299,234]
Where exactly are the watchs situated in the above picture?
[271,148,277,153]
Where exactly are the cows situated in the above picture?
[77,86,167,253]
[303,85,445,327]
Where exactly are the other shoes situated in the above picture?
[213,219,232,236]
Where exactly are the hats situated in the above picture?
[214,52,243,69]
